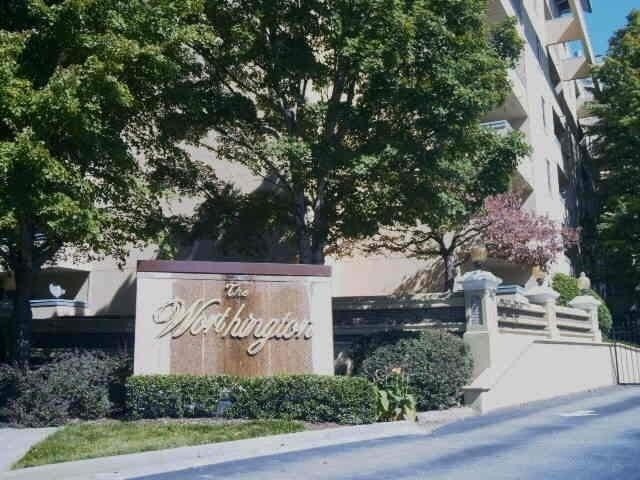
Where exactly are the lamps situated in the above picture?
[532,264,546,286]
[576,271,592,296]
[470,239,489,270]
